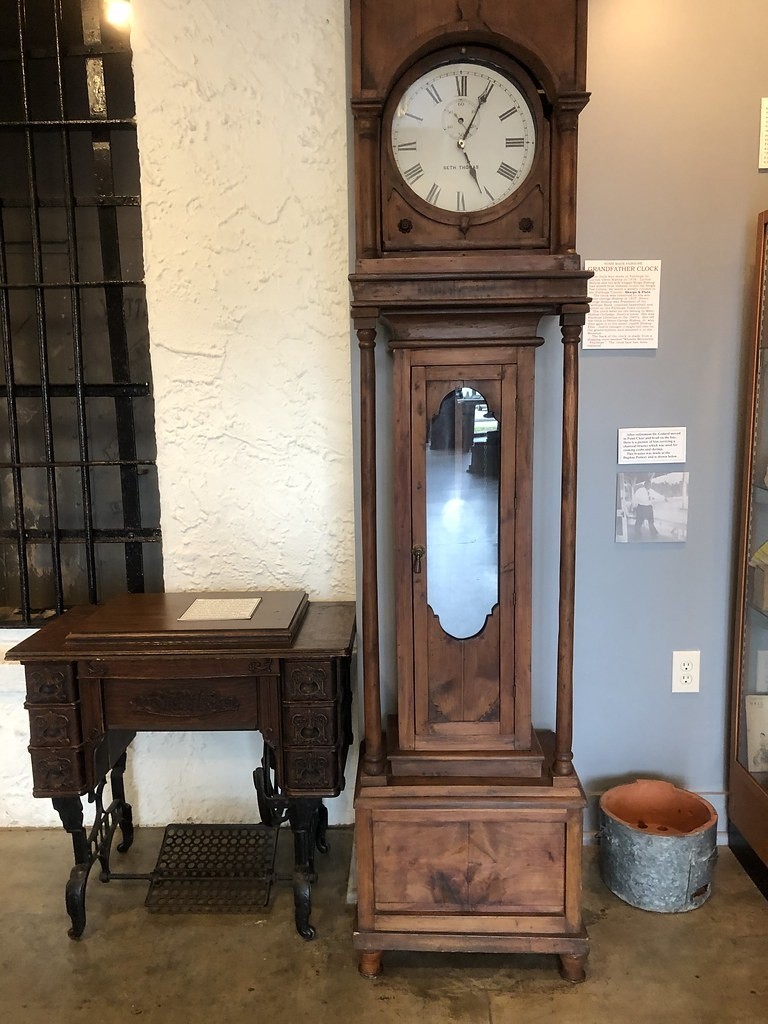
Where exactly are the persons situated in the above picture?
[630,479,669,537]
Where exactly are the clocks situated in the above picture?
[388,61,541,215]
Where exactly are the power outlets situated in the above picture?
[672,651,700,693]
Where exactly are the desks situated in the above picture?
[2,592,356,940]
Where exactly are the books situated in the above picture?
[744,692,768,774]
[178,596,262,620]
